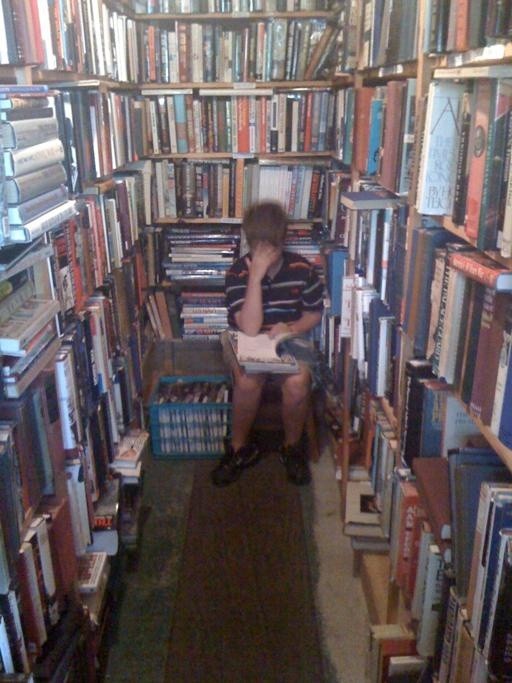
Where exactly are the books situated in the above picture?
[154,381,228,455]
[1,0,149,680]
[134,2,340,342]
[226,328,299,375]
[318,0,512,681]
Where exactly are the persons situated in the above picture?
[210,201,322,487]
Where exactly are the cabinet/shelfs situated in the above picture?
[136,0,331,439]
[333,2,512,681]
[2,0,144,673]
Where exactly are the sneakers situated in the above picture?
[210,431,260,487]
[275,432,310,486]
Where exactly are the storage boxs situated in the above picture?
[150,374,231,458]
